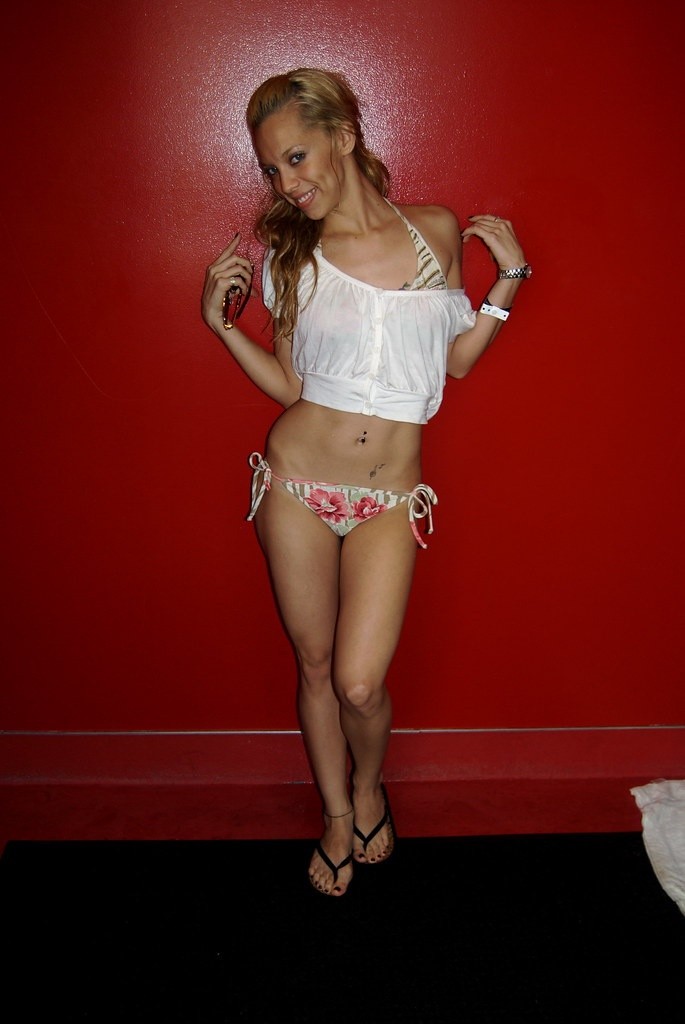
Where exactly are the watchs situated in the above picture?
[497,264,533,280]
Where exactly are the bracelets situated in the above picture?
[478,297,515,323]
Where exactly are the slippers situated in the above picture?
[351,782,397,868]
[308,832,354,897]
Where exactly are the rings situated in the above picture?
[229,276,237,287]
[493,217,502,222]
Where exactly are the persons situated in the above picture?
[202,69,532,899]
